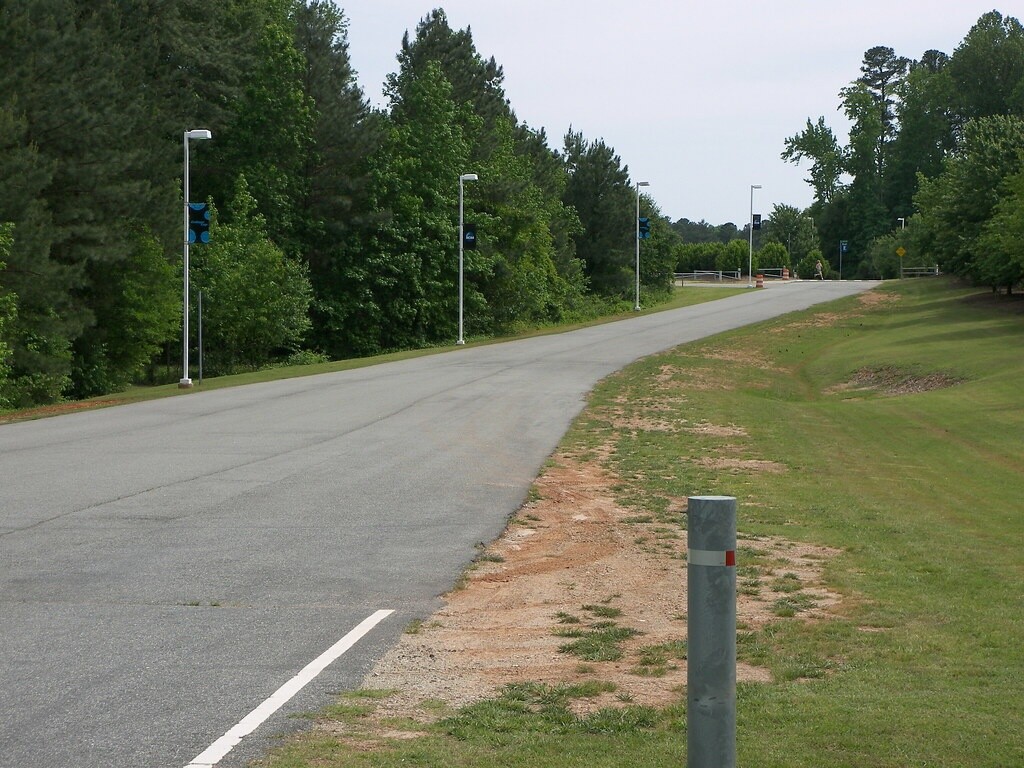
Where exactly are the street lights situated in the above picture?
[897,218,904,230]
[633,181,651,312]
[747,184,763,290]
[454,174,478,346]
[178,129,213,389]
